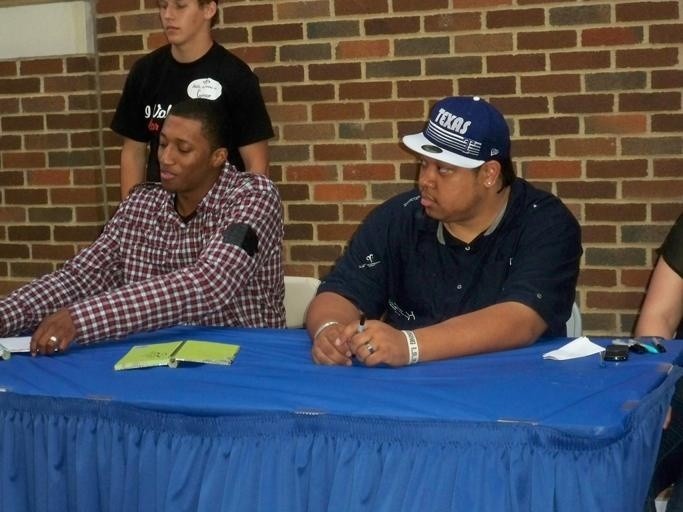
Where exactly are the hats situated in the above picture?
[402,95,510,169]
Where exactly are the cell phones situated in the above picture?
[604,345,628,361]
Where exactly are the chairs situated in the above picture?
[566,301,582,338]
[283,276,321,330]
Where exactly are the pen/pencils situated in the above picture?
[652,337,665,352]
[359,316,365,332]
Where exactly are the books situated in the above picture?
[114,342,240,371]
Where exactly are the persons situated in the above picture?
[302,93,584,369]
[0,98,285,360]
[633,213,682,511]
[109,0,274,203]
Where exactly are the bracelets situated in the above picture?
[313,320,338,341]
[401,330,419,366]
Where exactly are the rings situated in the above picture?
[48,336,57,344]
[365,343,373,354]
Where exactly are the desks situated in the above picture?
[0,324,683,511]
